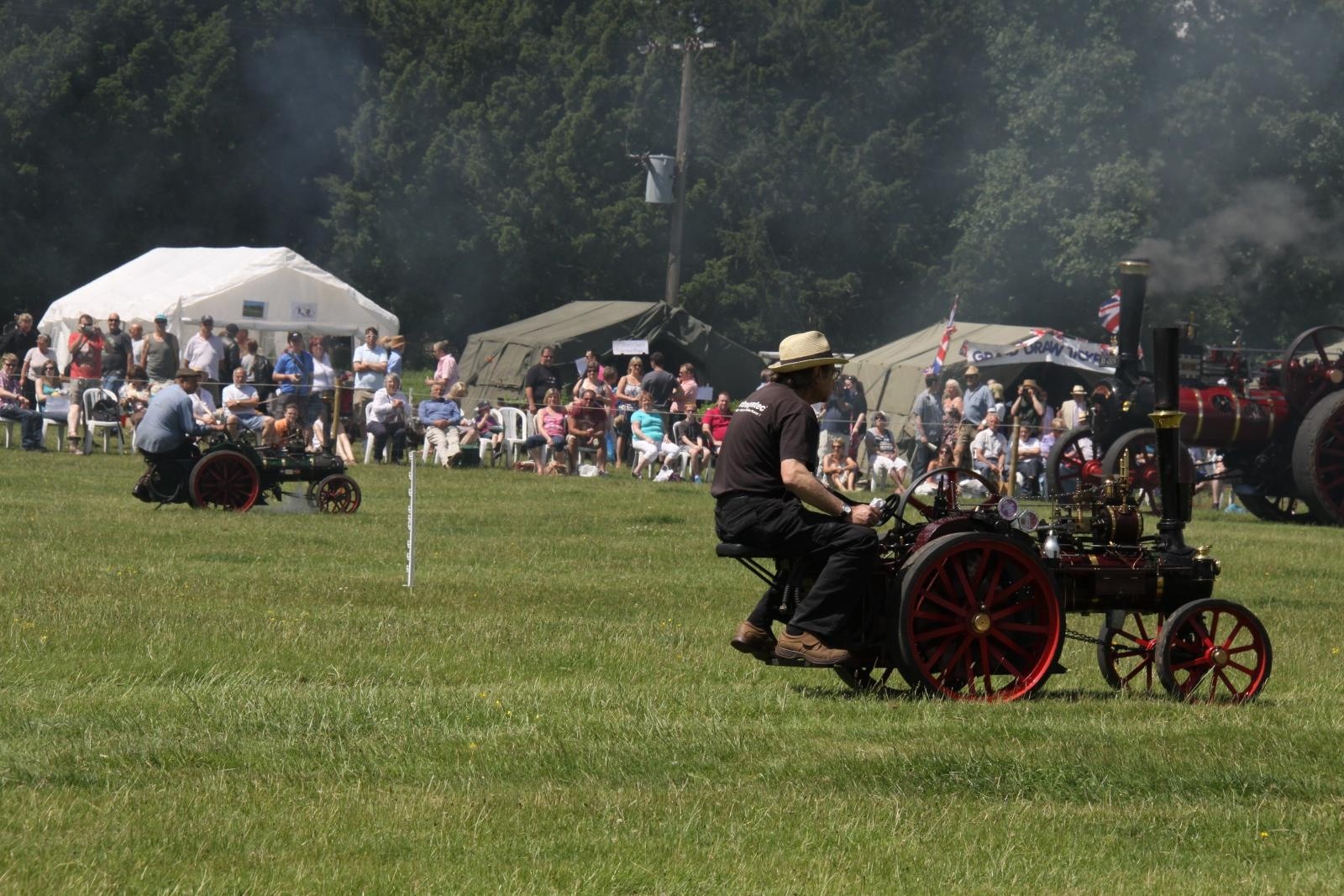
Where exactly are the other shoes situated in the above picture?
[21,430,91,457]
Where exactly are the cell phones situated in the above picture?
[82,326,96,332]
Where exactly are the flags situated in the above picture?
[925,303,958,377]
[1097,288,1145,360]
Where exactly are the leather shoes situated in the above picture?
[132,483,151,502]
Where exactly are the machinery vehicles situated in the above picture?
[715,259,1343,707]
[140,389,361,516]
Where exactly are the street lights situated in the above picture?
[642,11,719,308]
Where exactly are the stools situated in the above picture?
[714,540,814,613]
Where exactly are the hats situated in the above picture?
[1069,385,1087,396]
[477,400,492,414]
[1017,379,1042,393]
[175,368,201,378]
[389,335,407,350]
[964,365,981,376]
[767,330,850,374]
[874,410,891,427]
[1076,410,1089,422]
[2,296,308,353]
[989,383,1006,400]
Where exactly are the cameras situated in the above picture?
[1021,387,1031,393]
[837,389,850,396]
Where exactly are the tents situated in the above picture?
[839,317,1144,461]
[38,243,399,369]
[460,296,770,411]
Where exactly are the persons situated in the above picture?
[418,384,463,471]
[101,312,410,448]
[0,352,48,453]
[708,330,886,665]
[19,334,57,407]
[521,388,569,478]
[0,312,41,380]
[130,366,227,502]
[423,339,459,399]
[525,346,563,461]
[35,359,85,456]
[445,381,478,465]
[67,314,113,448]
[366,371,411,466]
[474,400,505,460]
[567,347,1266,510]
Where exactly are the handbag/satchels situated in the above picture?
[91,390,120,422]
[572,458,689,483]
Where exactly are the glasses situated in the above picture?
[875,415,885,420]
[685,409,696,413]
[584,398,594,402]
[832,445,842,449]
[3,359,61,374]
[827,368,841,380]
[632,363,642,367]
[540,351,600,377]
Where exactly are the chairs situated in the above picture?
[2,386,1048,500]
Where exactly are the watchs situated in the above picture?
[838,502,852,519]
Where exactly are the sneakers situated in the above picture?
[775,629,851,665]
[730,620,778,661]
